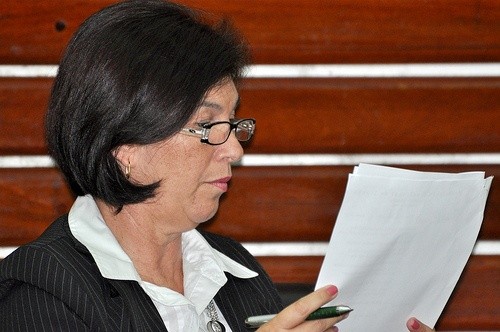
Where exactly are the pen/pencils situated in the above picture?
[244,305,354,327]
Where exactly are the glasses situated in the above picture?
[172,117,256,148]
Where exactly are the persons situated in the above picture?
[1,2,440,331]
